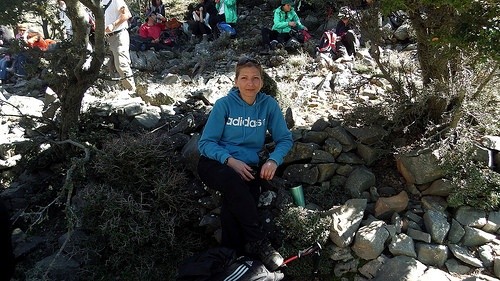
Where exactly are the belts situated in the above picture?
[104,29,122,38]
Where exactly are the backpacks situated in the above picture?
[215,22,236,38]
[316,30,338,53]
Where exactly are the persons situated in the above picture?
[0,0,384,91]
[197,56,293,273]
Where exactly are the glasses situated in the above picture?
[238,57,260,66]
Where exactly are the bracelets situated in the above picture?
[224,158,228,166]
[267,159,278,167]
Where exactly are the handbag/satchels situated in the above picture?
[159,28,188,47]
[289,27,304,43]
[390,10,406,28]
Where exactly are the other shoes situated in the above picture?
[262,249,284,270]
[237,264,284,281]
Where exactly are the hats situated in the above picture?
[281,0,294,6]
[145,9,156,22]
[338,6,356,19]
